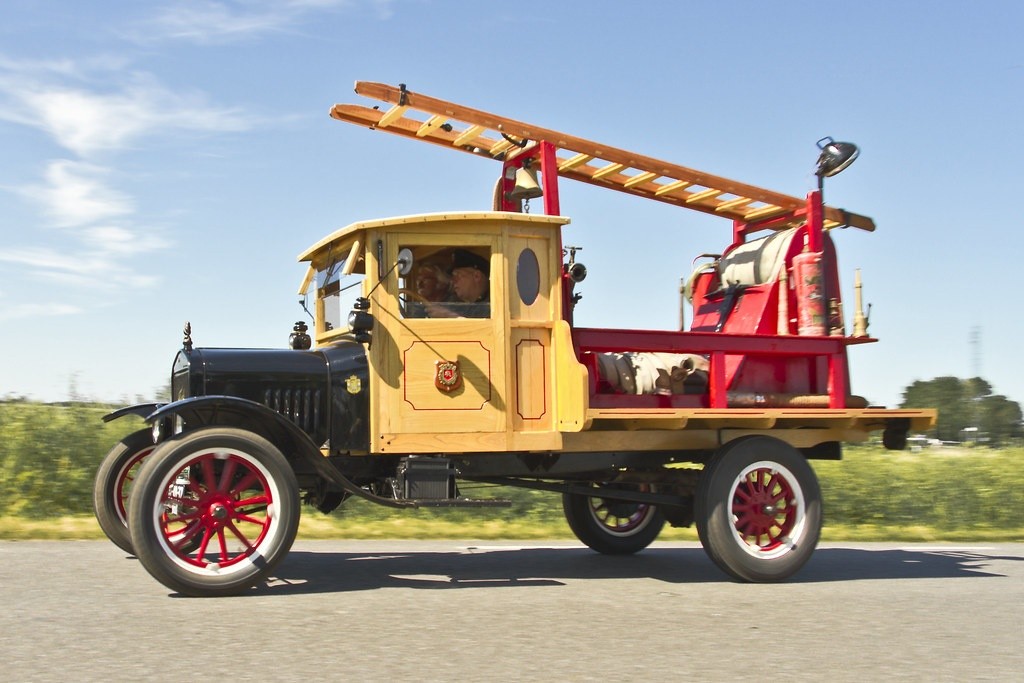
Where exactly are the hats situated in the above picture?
[448,251,490,273]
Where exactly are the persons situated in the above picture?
[424,248,490,318]
[407,261,450,318]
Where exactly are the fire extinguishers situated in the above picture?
[794,252,829,336]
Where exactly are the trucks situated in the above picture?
[92,80,941,598]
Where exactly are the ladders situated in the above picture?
[327,77,877,238]
